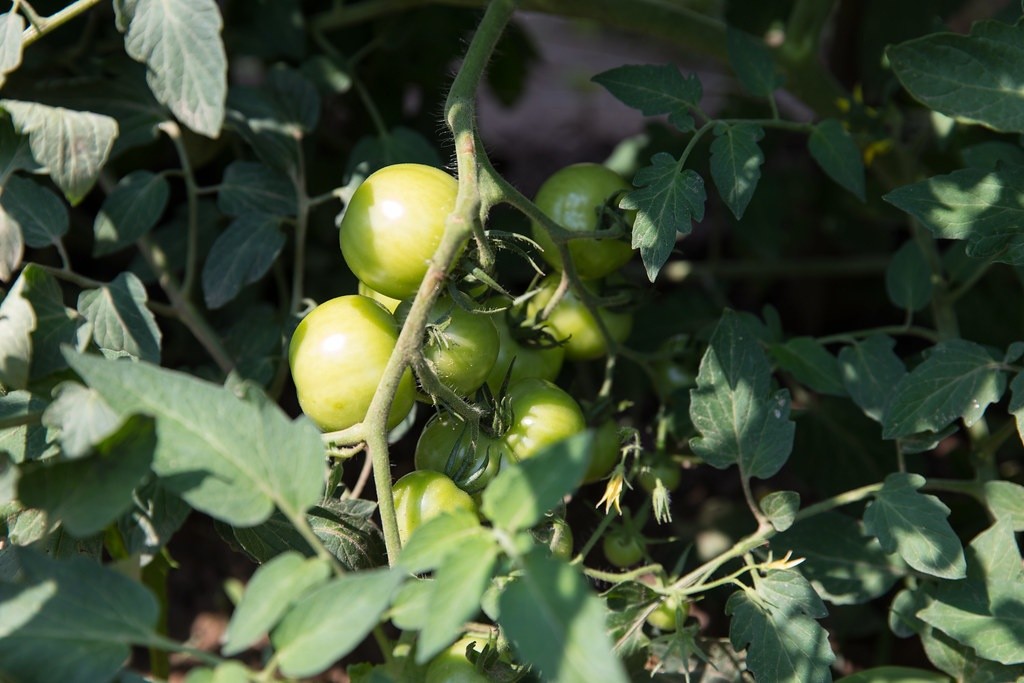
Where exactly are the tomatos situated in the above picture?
[290,163,702,683]
